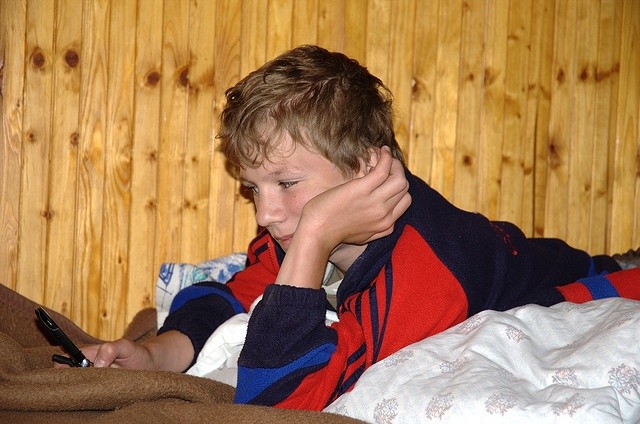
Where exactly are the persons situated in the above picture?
[53,44,639,411]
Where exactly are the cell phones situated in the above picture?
[34,305,94,368]
